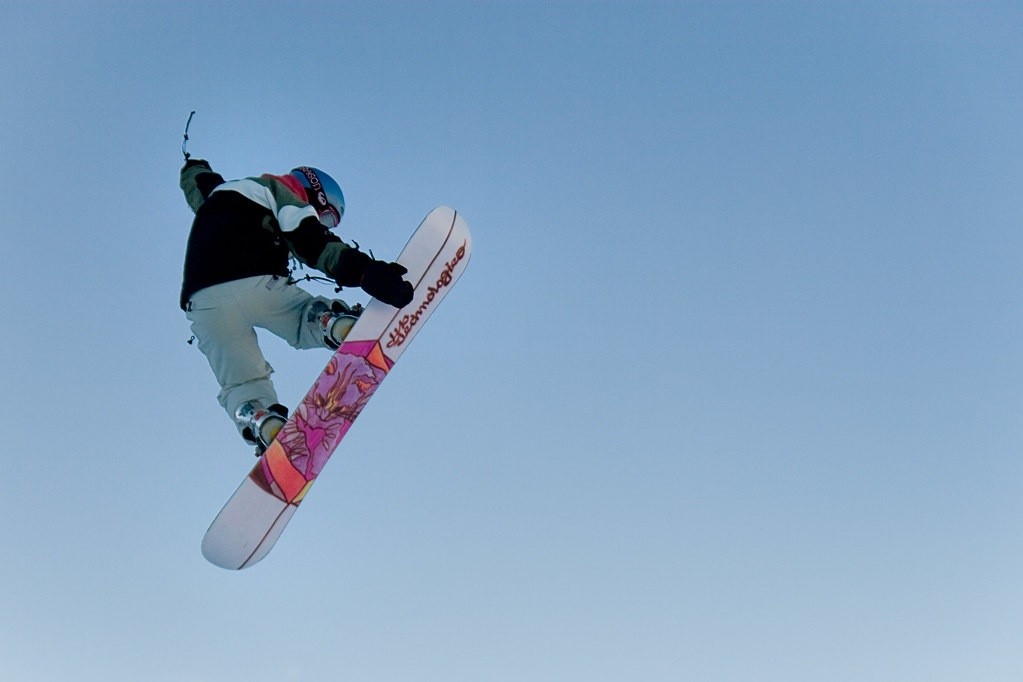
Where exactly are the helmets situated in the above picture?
[291,167,345,222]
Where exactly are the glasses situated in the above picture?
[318,207,340,229]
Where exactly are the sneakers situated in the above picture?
[234,400,288,449]
[305,300,359,347]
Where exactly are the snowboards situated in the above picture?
[199,206,474,572]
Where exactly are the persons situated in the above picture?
[179,160,414,458]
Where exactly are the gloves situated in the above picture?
[180,159,213,172]
[333,245,414,308]
